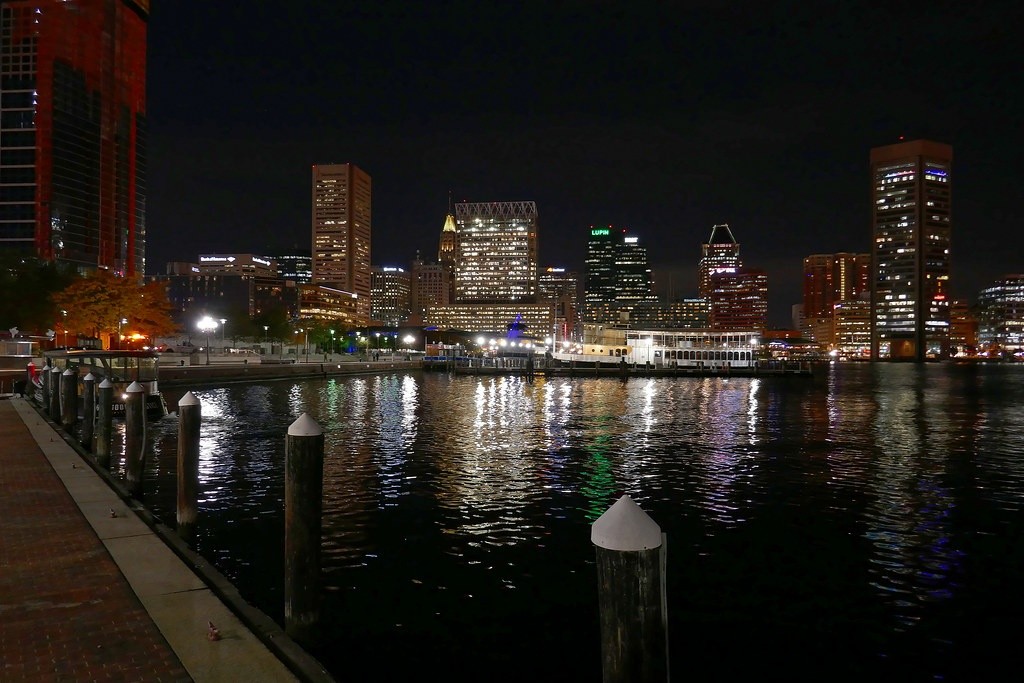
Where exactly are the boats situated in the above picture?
[550,321,761,368]
[33,349,170,420]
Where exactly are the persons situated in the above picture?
[372,352,380,361]
[323,351,328,362]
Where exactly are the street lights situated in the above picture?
[330,330,335,359]
[376,333,380,351]
[356,332,361,352]
[404,335,415,361]
[220,319,227,354]
[264,325,269,354]
[198,317,218,365]
